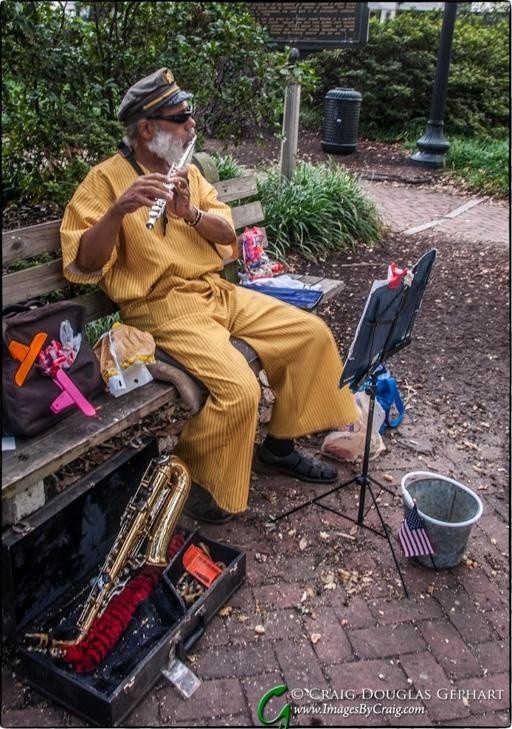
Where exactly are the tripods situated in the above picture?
[271,372,409,597]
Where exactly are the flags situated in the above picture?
[397,505,436,559]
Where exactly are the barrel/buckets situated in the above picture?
[399,470,485,572]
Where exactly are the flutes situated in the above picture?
[146,135,197,230]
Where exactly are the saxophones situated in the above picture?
[20,455,191,659]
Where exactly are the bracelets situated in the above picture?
[185,208,204,228]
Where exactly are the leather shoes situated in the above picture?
[256,443,337,482]
[181,488,233,523]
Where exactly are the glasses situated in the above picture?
[148,107,194,124]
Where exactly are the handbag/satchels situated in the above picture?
[0,299,106,441]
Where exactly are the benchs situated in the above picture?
[2,152,344,548]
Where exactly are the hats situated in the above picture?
[117,68,194,120]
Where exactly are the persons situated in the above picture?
[63,65,362,525]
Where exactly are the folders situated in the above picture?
[337,249,437,389]
